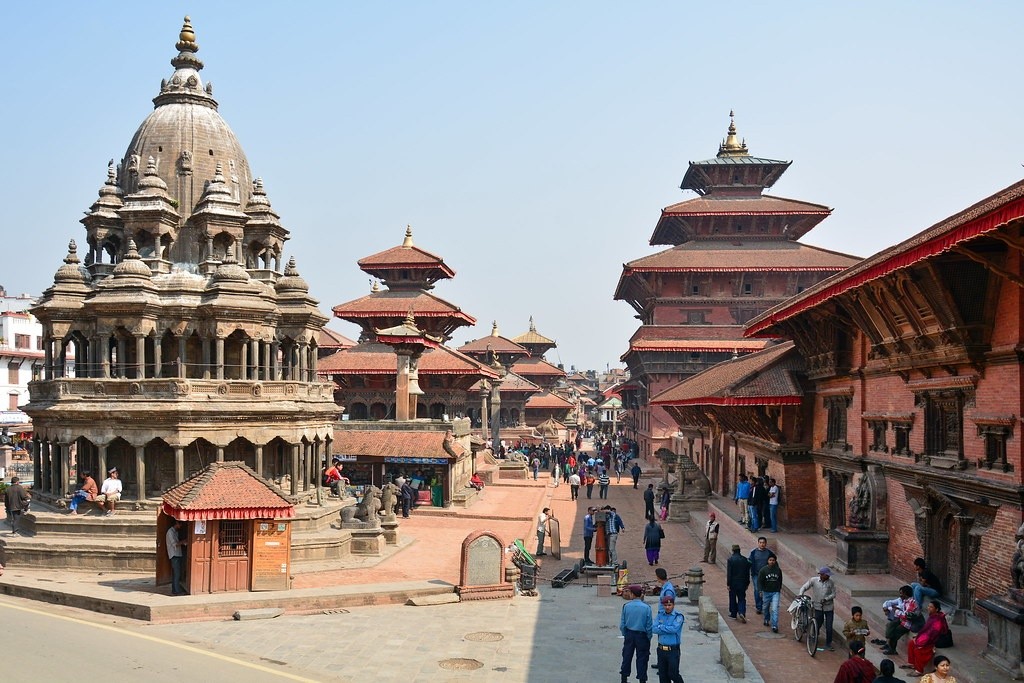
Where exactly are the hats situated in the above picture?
[732,544,740,551]
[818,568,834,575]
[661,595,674,604]
[628,584,642,592]
[709,512,715,517]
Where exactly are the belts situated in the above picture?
[659,644,679,650]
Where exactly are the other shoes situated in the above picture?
[812,634,819,642]
[883,649,898,655]
[763,620,769,626]
[907,670,925,677]
[756,609,761,614]
[709,562,715,564]
[772,626,778,633]
[651,664,658,668]
[899,663,914,669]
[739,614,746,623]
[700,560,708,563]
[871,638,887,645]
[727,614,737,620]
[825,645,835,651]
[879,644,889,650]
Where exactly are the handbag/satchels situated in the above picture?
[887,606,899,622]
[935,629,954,649]
[911,614,926,632]
[659,525,665,538]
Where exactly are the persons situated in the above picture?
[871,659,907,683]
[842,606,870,659]
[536,508,552,556]
[65,469,98,515]
[726,544,752,623]
[617,585,652,683]
[874,584,926,656]
[467,413,643,502]
[910,557,943,613]
[651,595,685,683]
[611,507,625,534]
[919,655,958,683]
[94,467,123,518]
[4,476,31,538]
[644,483,658,522]
[602,505,621,568]
[583,506,599,566]
[733,472,780,533]
[166,520,189,595]
[643,514,665,566]
[699,512,720,563]
[799,568,836,652]
[833,640,877,683]
[758,553,784,633]
[659,486,670,520]
[320,457,437,520]
[747,536,774,615]
[650,568,676,675]
[15,438,78,478]
[899,600,950,677]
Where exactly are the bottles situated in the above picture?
[853,628,869,635]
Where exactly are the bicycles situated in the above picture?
[790,594,824,656]
